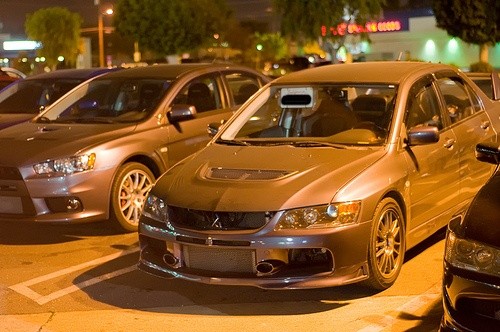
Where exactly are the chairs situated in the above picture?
[239,83,258,103]
[352,95,387,122]
[187,83,215,113]
[136,83,164,114]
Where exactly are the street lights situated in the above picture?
[97,0,114,68]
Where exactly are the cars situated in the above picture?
[442,144,499,332]
[0,63,284,233]
[137,60,500,292]
[0,66,129,131]
